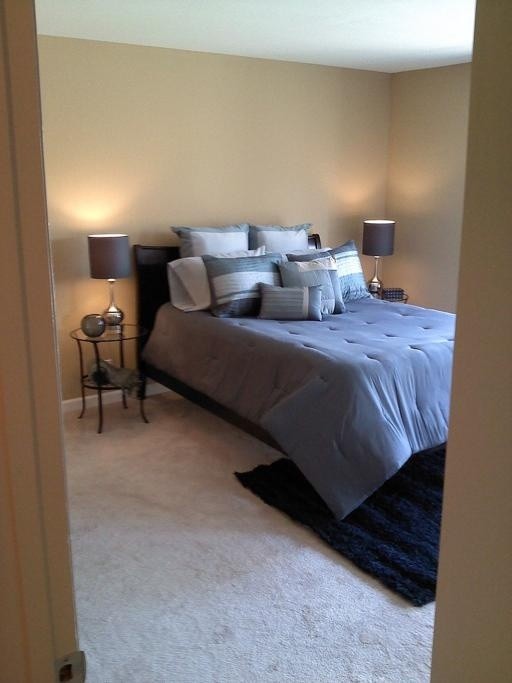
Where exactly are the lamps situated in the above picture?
[362,219,395,292]
[88,233,132,325]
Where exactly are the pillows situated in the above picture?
[167,223,375,320]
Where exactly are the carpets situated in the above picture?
[233,443,447,608]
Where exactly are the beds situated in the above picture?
[134,233,457,492]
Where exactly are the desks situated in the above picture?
[70,323,157,433]
[372,292,408,303]
